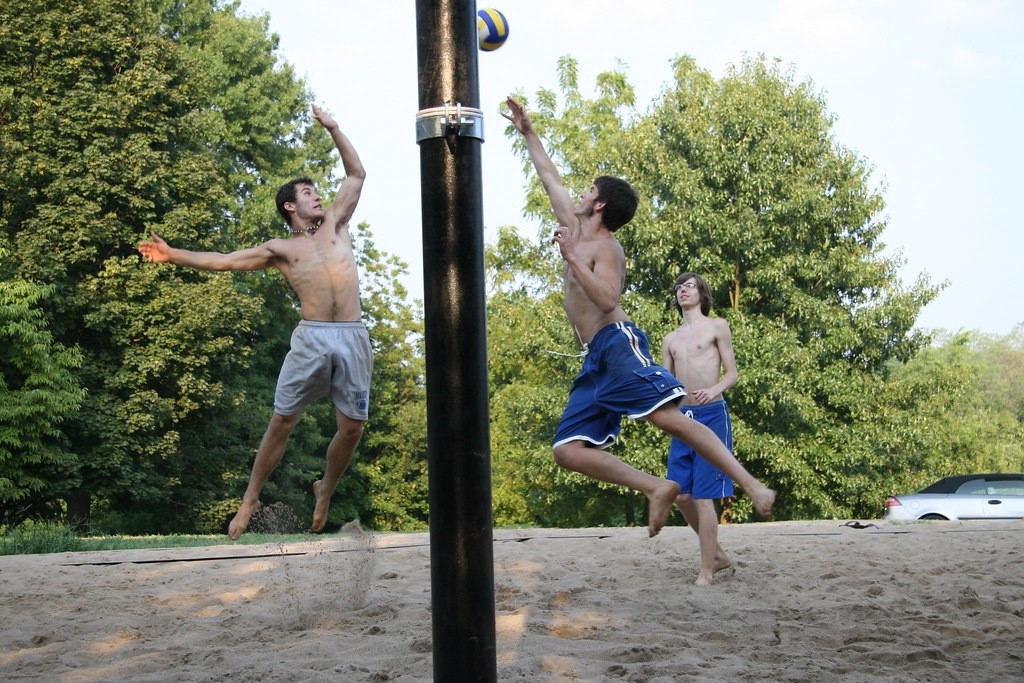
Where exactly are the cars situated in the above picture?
[882,473,1024,524]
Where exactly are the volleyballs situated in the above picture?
[478,7,509,52]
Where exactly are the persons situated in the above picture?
[659,272,740,587]
[137,104,373,540]
[498,95,779,540]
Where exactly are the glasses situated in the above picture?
[675,283,698,291]
